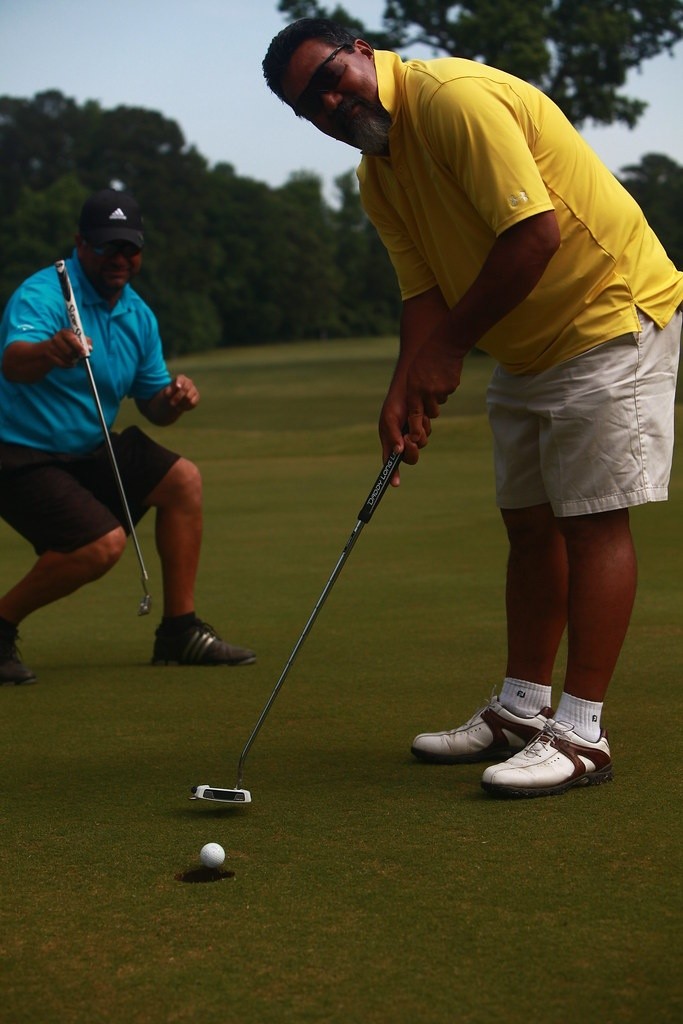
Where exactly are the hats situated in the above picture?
[78,189,146,246]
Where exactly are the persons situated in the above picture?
[261,19,683,798]
[0,192,257,688]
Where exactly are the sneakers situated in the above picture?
[412,696,552,762]
[482,720,615,797]
[0,623,35,684]
[152,621,257,665]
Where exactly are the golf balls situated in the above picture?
[201,843,226,868]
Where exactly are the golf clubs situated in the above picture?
[186,448,399,805]
[54,259,154,614]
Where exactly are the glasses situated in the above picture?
[294,45,353,119]
[79,238,142,256]
